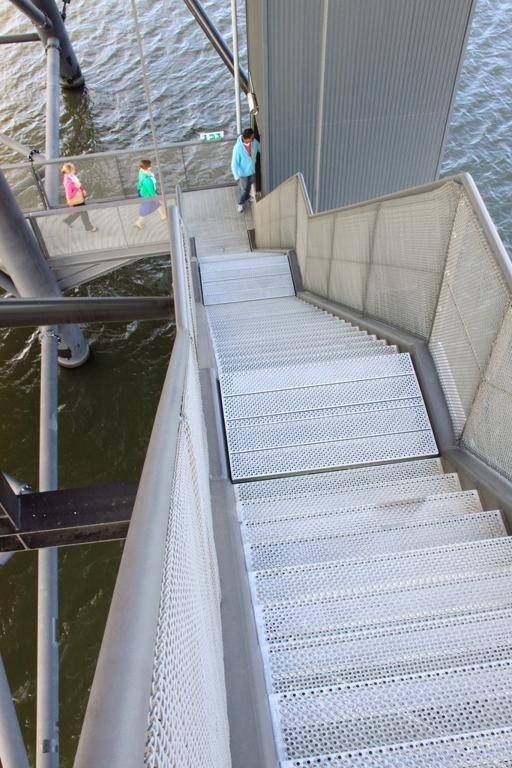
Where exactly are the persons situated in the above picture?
[63,161,99,232]
[132,159,167,229]
[230,128,262,214]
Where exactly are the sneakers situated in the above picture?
[237,204,244,212]
[61,219,98,233]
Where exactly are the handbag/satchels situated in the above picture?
[67,190,84,205]
